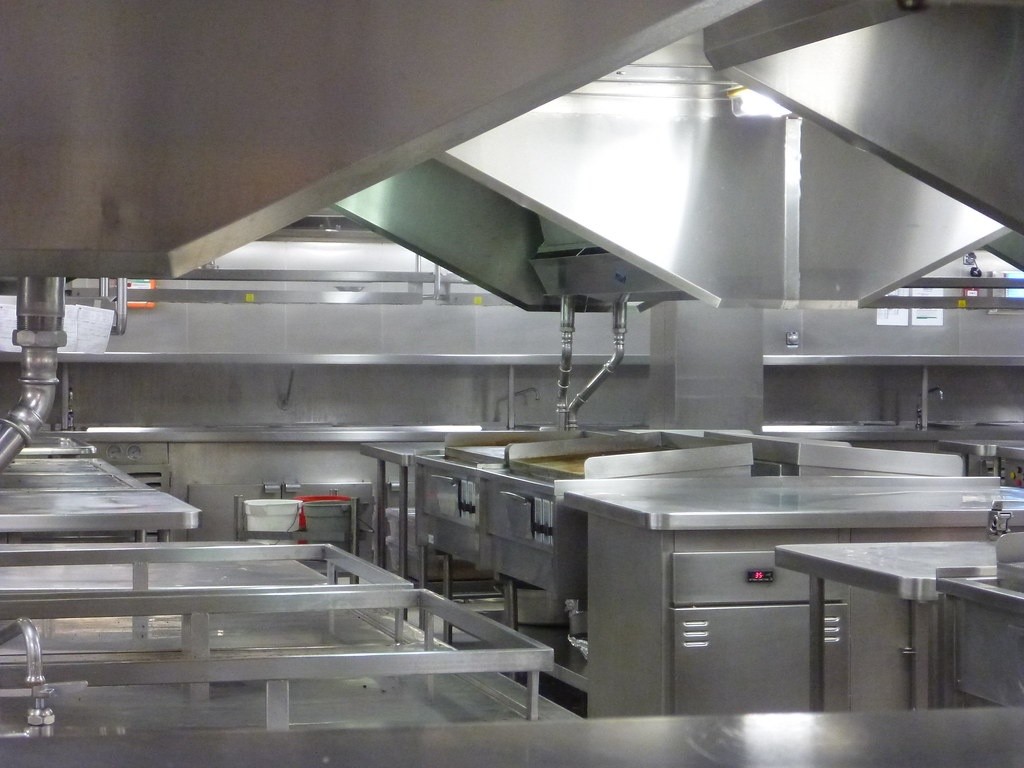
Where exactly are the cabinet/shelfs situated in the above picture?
[359,429,1024,719]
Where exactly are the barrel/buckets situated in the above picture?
[242,495,351,546]
